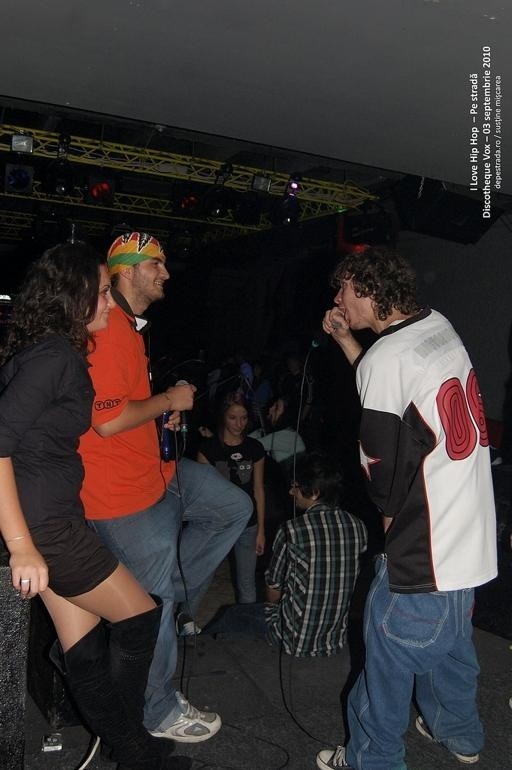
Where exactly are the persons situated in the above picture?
[78,230,255,744]
[0,240,192,770]
[316,244,499,770]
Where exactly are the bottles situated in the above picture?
[154,410,174,462]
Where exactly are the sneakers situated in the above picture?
[315,748,356,770]
[415,714,480,764]
[176,612,203,638]
[147,691,222,743]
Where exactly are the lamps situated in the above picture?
[251,174,273,193]
[9,133,35,154]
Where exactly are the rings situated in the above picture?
[21,580,30,583]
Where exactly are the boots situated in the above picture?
[49,593,194,770]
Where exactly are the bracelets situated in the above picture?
[6,533,31,543]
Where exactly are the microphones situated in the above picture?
[312,311,342,348]
[174,380,190,433]
